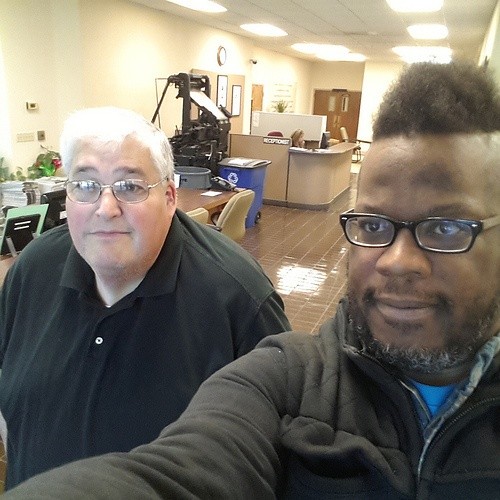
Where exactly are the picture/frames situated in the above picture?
[231,85,241,116]
[216,75,228,108]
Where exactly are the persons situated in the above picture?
[291,129,306,148]
[1,104,292,493]
[3,63,500,500]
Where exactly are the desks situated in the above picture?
[0,188,238,284]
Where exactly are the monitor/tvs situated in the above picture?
[320,132,331,149]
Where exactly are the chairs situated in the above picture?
[185,189,255,240]
[340,126,361,163]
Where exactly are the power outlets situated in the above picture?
[37,131,45,140]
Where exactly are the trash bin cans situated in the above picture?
[218,157,272,228]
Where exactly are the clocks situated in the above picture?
[218,46,226,65]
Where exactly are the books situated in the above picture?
[1,177,67,209]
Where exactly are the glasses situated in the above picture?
[339,208,500,253]
[63,172,169,204]
